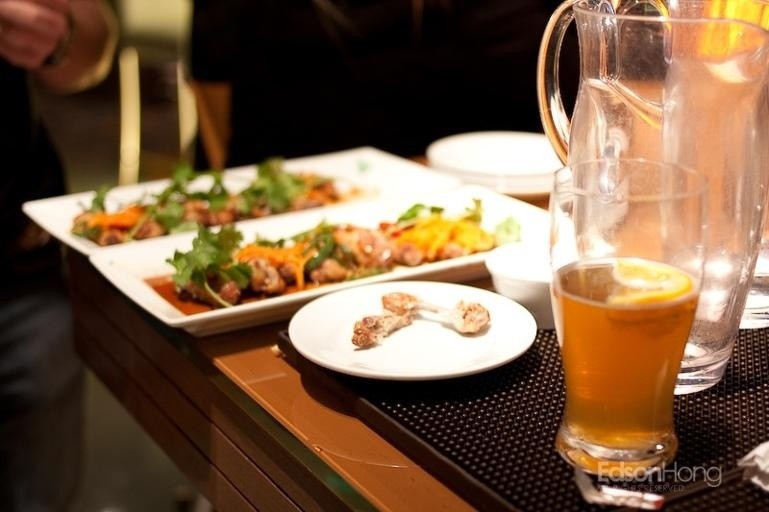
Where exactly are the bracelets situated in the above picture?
[42,6,81,73]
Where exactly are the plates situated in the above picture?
[20,132,565,383]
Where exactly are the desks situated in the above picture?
[69,157,769,512]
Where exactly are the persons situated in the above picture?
[2,2,124,504]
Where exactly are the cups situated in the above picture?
[545,155,708,475]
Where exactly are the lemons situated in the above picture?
[608,258,693,304]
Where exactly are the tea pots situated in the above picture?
[536,0,769,395]
